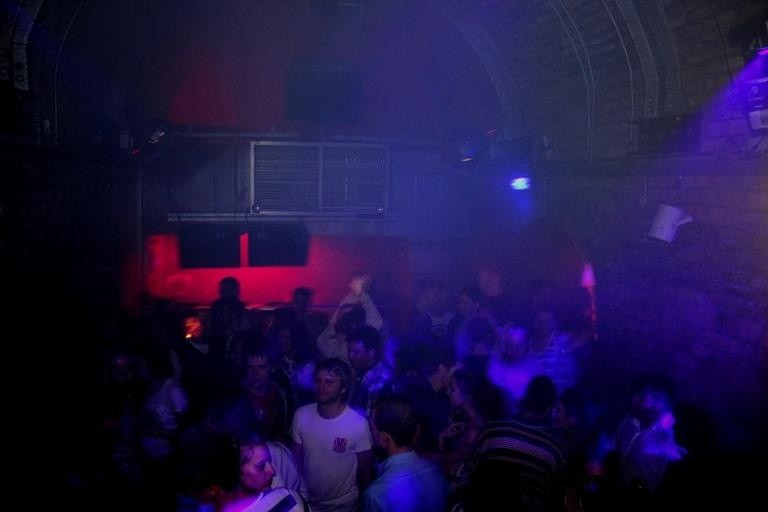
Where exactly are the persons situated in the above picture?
[1,270,768,512]
